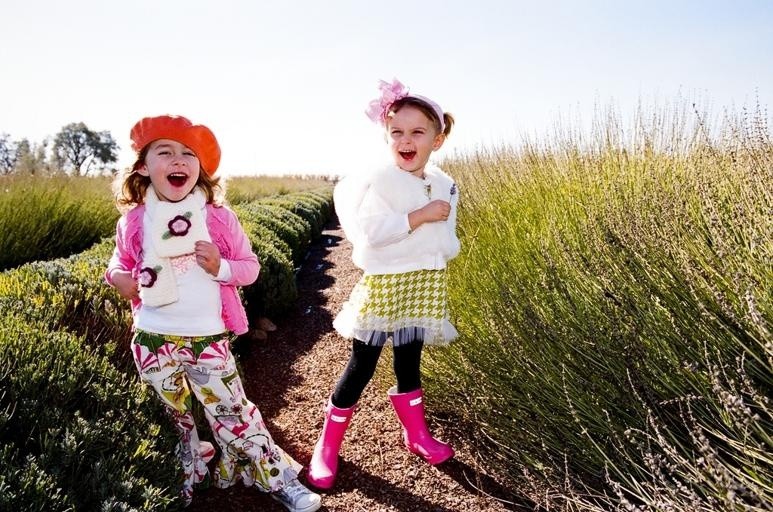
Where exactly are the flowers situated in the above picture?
[448,183,456,204]
[138,265,162,289]
[161,211,192,241]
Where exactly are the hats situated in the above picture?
[131,113,220,179]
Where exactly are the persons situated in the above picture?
[104,115,321,511]
[303,76,461,492]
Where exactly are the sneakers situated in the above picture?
[272,480,321,511]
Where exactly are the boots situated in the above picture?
[386,386,456,464]
[306,393,358,491]
